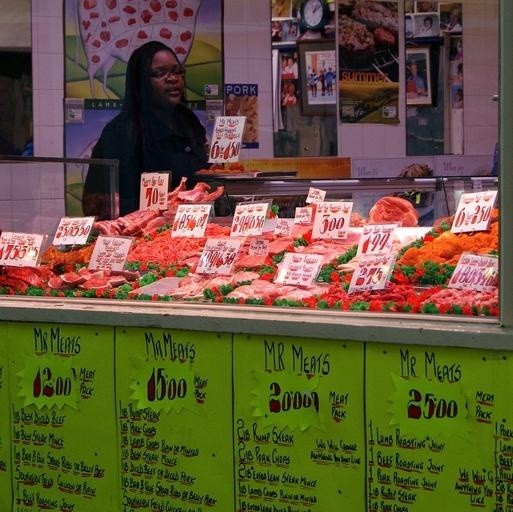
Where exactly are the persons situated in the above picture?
[272,20,336,106]
[82,40,210,221]
[406,1,462,96]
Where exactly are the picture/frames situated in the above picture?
[296,39,337,116]
[270,15,301,45]
[404,45,434,107]
[404,1,462,37]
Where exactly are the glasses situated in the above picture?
[155,67,185,81]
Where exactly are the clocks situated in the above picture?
[301,0,330,30]
